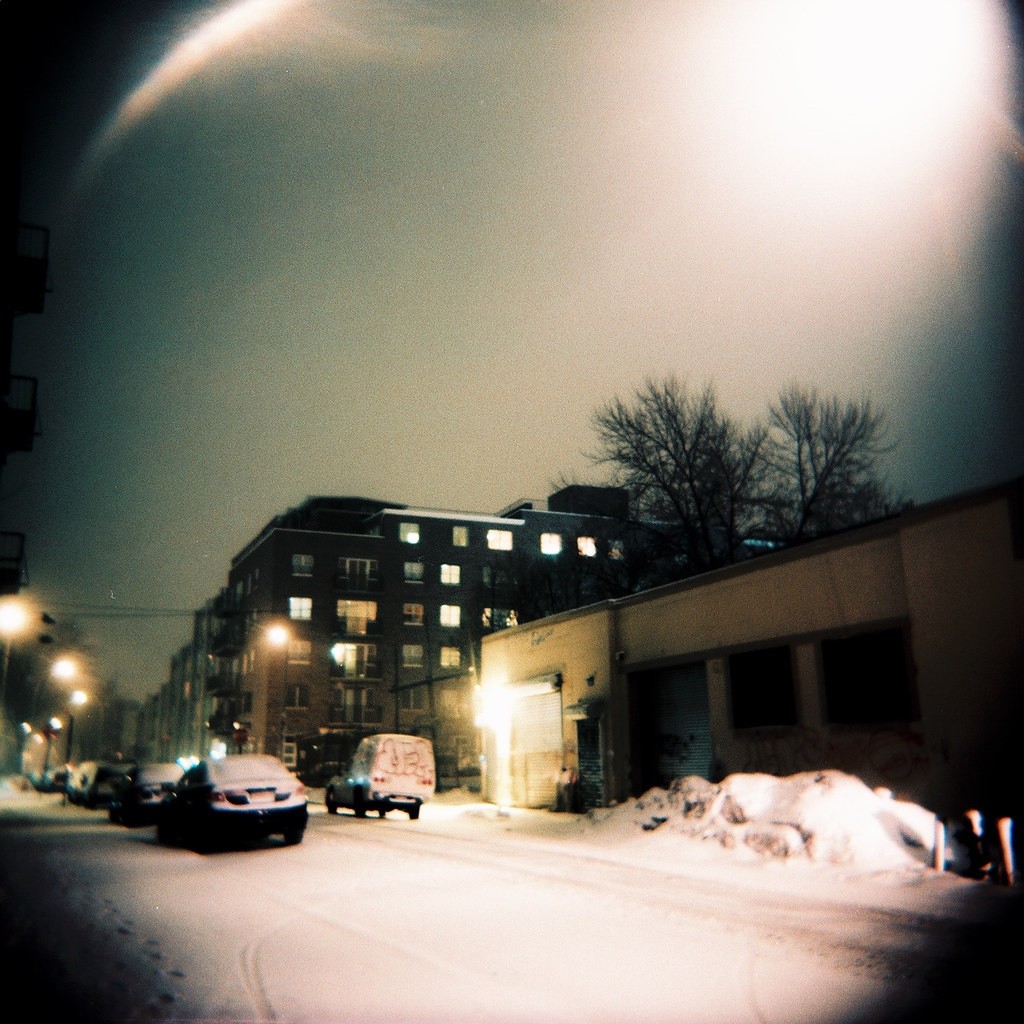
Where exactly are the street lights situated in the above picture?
[43,656,75,767]
[65,688,87,762]
[0,595,35,694]
[269,622,288,753]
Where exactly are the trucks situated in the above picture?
[322,733,436,821]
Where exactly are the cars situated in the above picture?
[108,763,187,829]
[157,755,310,849]
[42,765,68,793]
[438,765,482,792]
[66,762,126,808]
[299,762,345,786]
